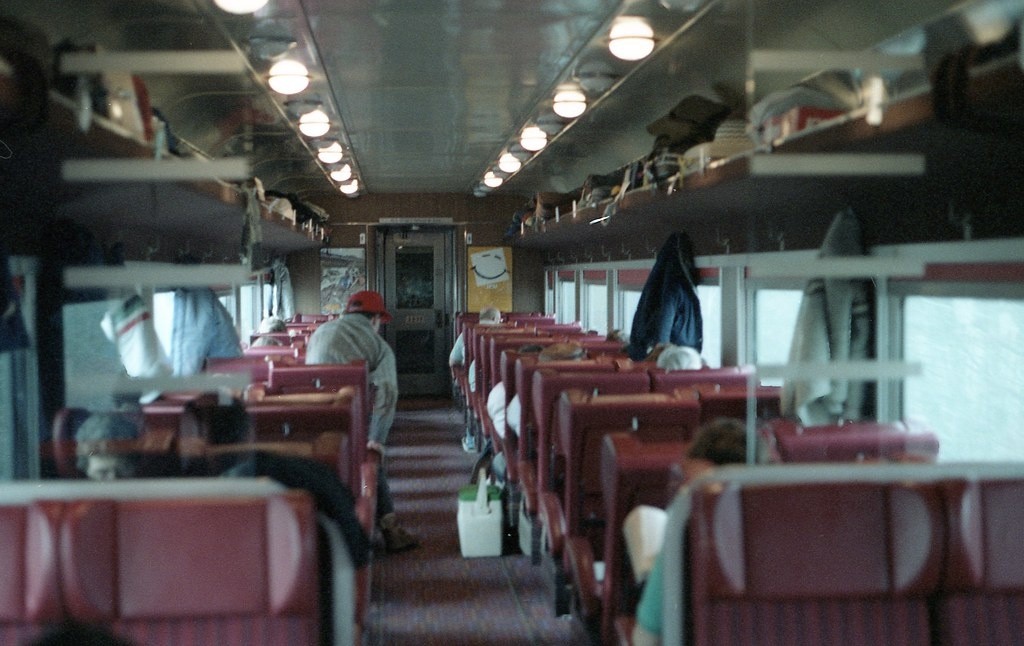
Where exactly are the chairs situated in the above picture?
[451,307,1023,646]
[0,315,380,645]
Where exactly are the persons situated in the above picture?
[462,331,703,479]
[75,414,149,482]
[449,306,500,381]
[305,292,426,553]
[630,419,774,646]
[178,392,371,574]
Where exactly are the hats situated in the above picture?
[538,342,587,363]
[260,317,287,336]
[657,346,703,371]
[347,289,392,324]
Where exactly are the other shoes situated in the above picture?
[382,512,420,552]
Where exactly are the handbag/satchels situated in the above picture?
[457,467,502,558]
